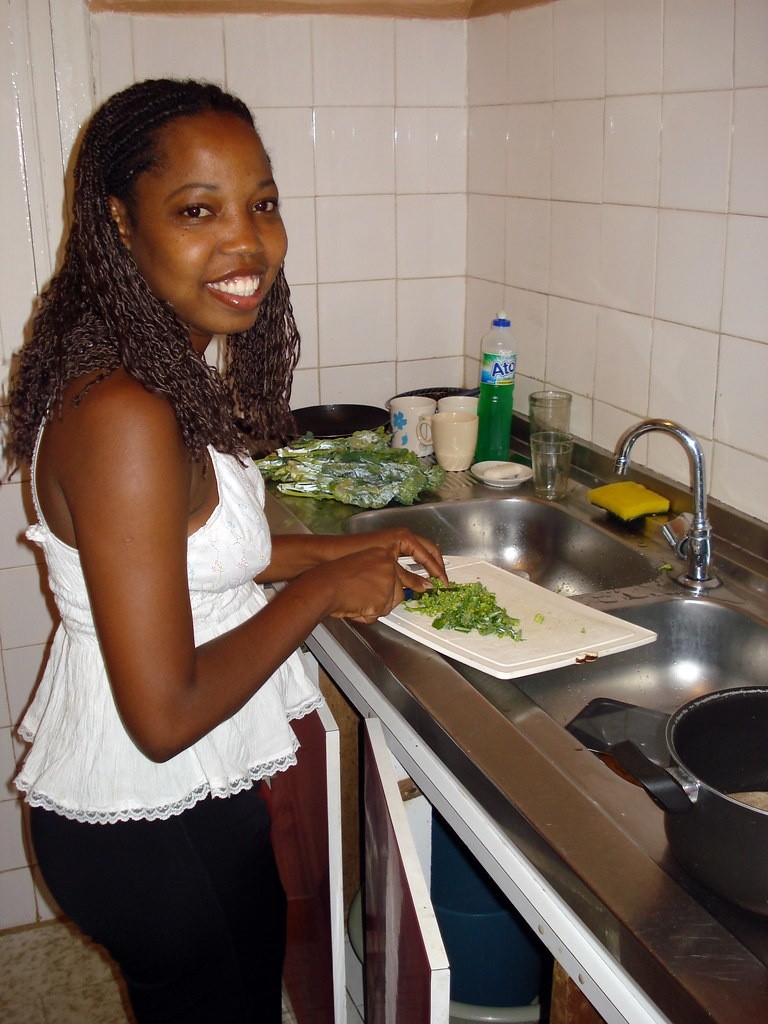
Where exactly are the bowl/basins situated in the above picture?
[348,885,542,1024]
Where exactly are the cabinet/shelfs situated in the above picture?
[255,580,677,1024]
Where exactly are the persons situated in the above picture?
[3,79,449,1024]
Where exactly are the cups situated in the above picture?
[387,395,434,456]
[417,412,480,472]
[533,444,572,499]
[531,431,574,456]
[437,395,478,413]
[529,390,573,432]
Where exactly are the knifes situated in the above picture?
[404,586,463,602]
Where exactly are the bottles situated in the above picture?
[474,312,515,461]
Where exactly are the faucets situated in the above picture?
[611,417,713,582]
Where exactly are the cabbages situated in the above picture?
[256,425,445,510]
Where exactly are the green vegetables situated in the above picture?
[402,577,525,641]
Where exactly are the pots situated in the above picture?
[385,387,481,410]
[612,685,768,917]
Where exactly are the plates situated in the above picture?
[288,403,391,439]
[471,460,532,487]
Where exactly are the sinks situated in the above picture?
[509,595,768,789]
[338,494,667,598]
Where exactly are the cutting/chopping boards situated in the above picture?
[390,554,656,681]
